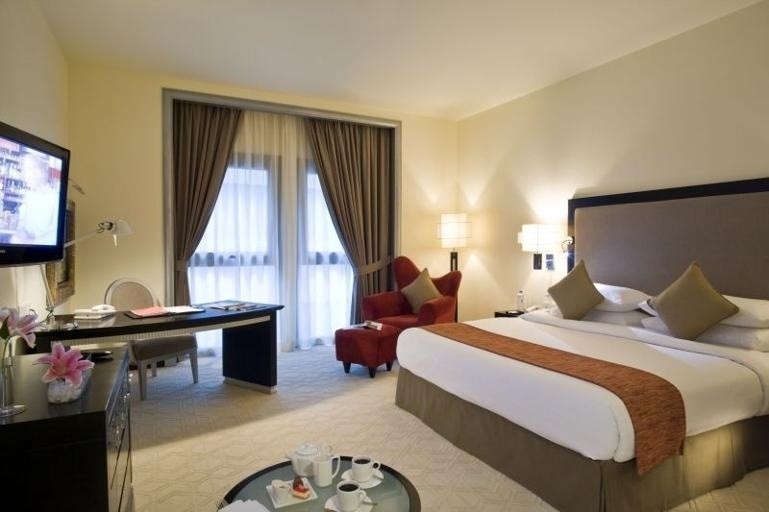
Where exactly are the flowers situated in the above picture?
[0,306,41,353]
[31,341,96,387]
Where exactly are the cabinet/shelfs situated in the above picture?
[1,346,136,512]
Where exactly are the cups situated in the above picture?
[336,479,366,512]
[351,454,381,483]
[309,453,340,487]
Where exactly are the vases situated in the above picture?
[47,364,92,403]
[0,340,26,417]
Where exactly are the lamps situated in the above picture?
[39,217,135,328]
[518,222,556,271]
[439,212,469,323]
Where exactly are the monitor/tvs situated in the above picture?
[0,121,70,268]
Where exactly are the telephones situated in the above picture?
[73,304,115,317]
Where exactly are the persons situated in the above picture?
[10,153,59,245]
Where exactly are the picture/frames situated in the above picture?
[45,200,76,309]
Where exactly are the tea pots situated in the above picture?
[285,442,333,477]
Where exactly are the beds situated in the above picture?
[395,177,769,511]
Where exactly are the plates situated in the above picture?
[264,477,318,507]
[324,493,374,512]
[341,468,384,489]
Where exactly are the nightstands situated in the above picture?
[494,310,525,317]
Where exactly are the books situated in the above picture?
[212,301,262,312]
[353,319,383,330]
[125,304,205,318]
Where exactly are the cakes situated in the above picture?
[291,476,310,500]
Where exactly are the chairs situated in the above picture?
[104,279,200,400]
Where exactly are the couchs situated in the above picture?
[359,255,463,358]
[334,323,402,377]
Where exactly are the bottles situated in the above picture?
[516,291,525,311]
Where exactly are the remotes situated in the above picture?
[81,350,112,356]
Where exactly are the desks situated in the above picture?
[22,299,285,394]
[215,456,422,511]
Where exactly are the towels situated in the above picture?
[638,291,769,331]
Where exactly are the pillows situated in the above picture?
[590,280,652,314]
[640,315,769,352]
[646,261,740,339]
[547,306,647,329]
[400,267,443,314]
[547,258,605,320]
